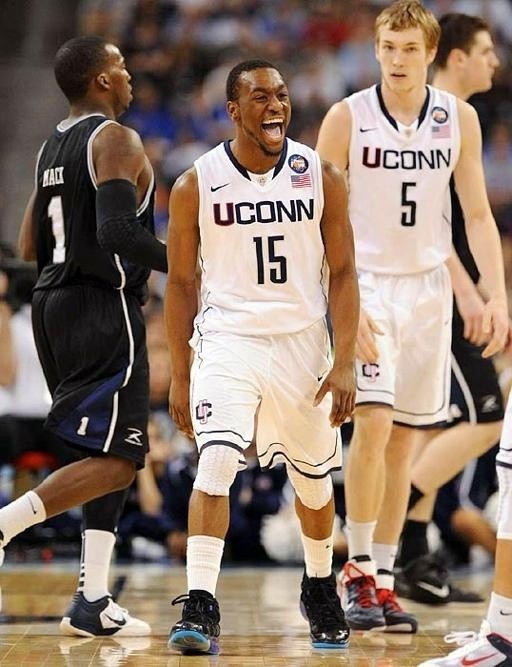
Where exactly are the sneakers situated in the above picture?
[375,588,419,633]
[392,557,488,612]
[167,589,221,655]
[417,619,512,667]
[299,566,351,650]
[58,590,152,637]
[338,562,388,633]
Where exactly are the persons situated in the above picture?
[0,32,203,638]
[404,388,512,667]
[163,56,363,655]
[59,638,158,666]
[363,633,419,652]
[312,0,512,634]
[0,1,511,607]
